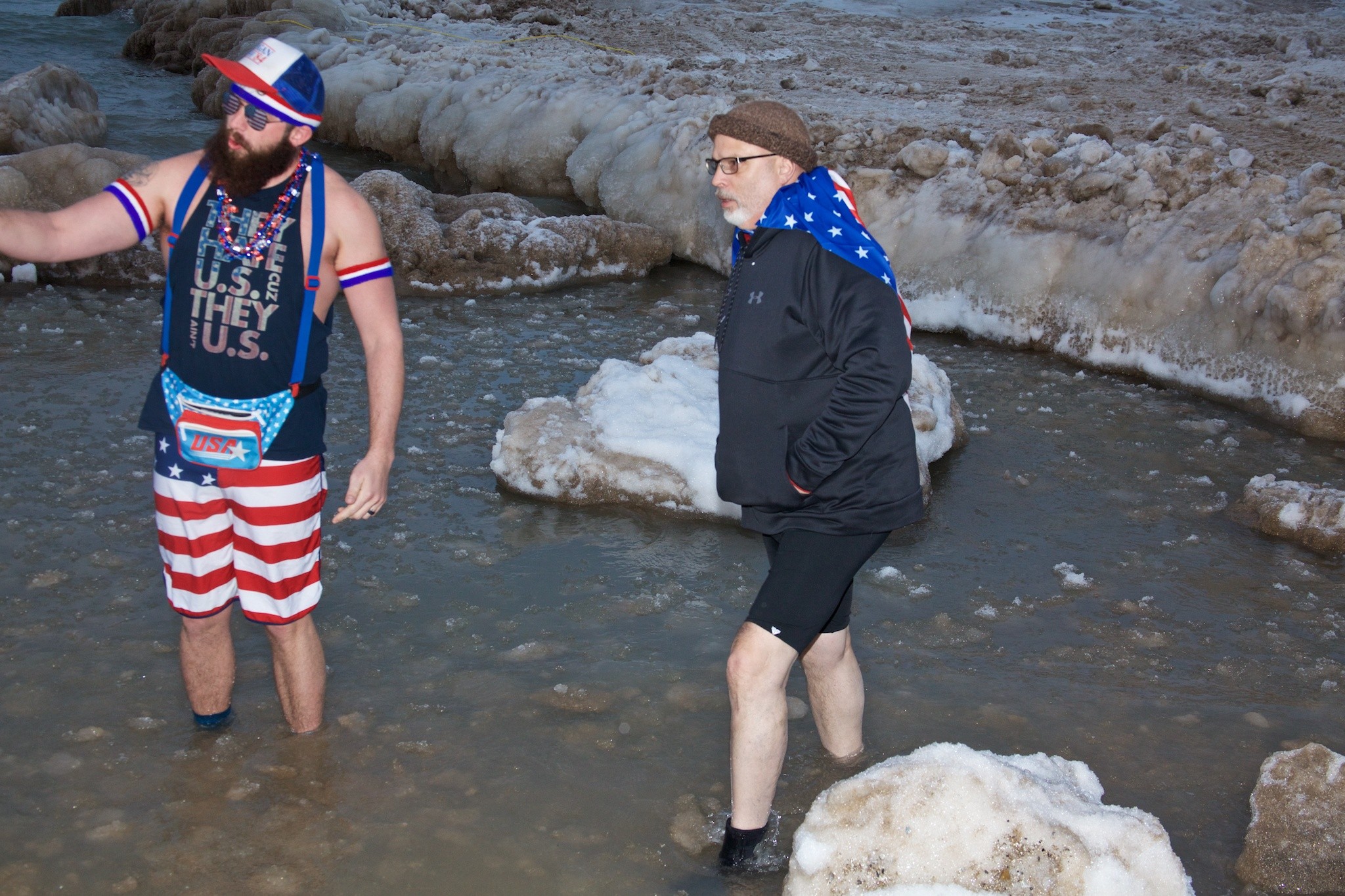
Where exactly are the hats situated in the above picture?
[201,36,325,132]
[708,101,818,174]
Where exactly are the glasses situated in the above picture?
[219,93,284,131]
[707,152,777,175]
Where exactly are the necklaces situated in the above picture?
[215,149,318,259]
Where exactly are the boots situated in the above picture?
[674,815,769,896]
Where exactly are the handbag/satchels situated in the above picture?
[161,367,297,470]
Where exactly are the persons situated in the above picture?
[705,102,922,868]
[0,35,402,735]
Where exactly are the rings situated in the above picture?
[368,511,375,515]
